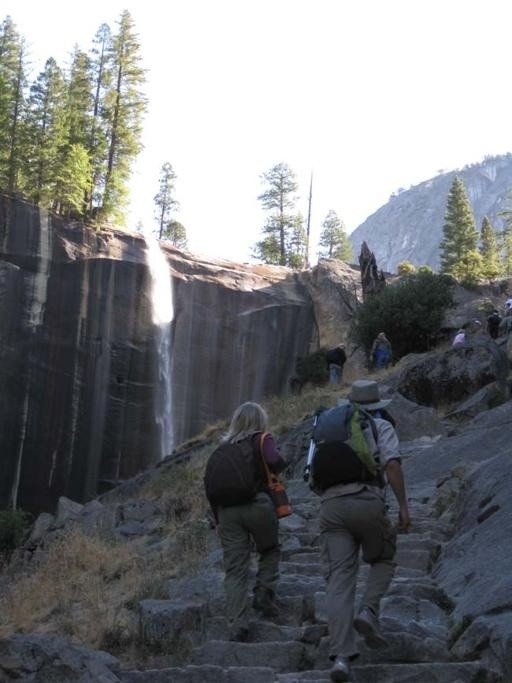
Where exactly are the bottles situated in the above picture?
[271,476,293,519]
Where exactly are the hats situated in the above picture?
[337,380,393,411]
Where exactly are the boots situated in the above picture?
[330,660,355,683]
[230,619,250,641]
[252,586,280,617]
[353,606,390,651]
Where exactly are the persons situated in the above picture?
[453,325,467,345]
[505,297,512,315]
[488,310,503,338]
[327,344,347,384]
[365,330,393,369]
[308,377,411,680]
[203,400,288,640]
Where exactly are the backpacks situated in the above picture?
[307,402,387,496]
[206,431,261,507]
